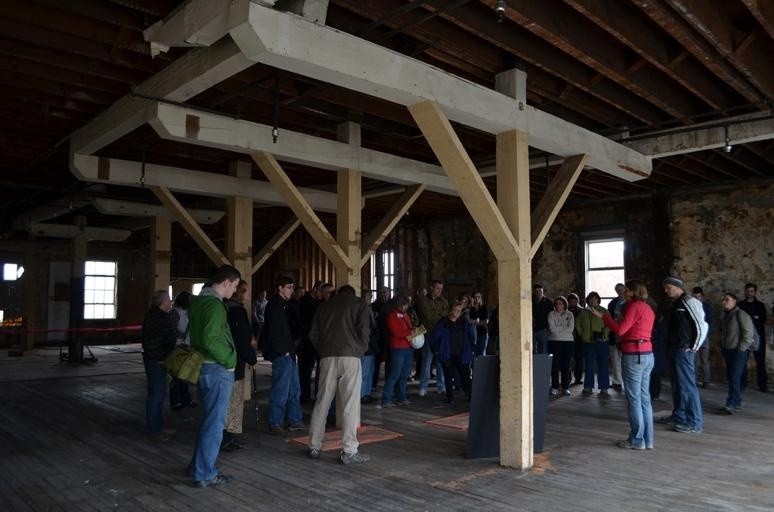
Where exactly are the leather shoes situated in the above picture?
[220,432,247,452]
[185,465,219,474]
[194,475,230,487]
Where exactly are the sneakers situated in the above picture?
[703,381,711,388]
[617,439,653,449]
[171,401,198,410]
[724,403,742,413]
[661,415,703,433]
[550,378,622,397]
[148,431,174,441]
[309,449,320,458]
[759,385,769,392]
[339,452,369,464]
[271,422,309,432]
[361,373,455,408]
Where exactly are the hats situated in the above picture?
[663,275,683,288]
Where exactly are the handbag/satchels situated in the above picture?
[749,323,761,351]
[166,344,202,385]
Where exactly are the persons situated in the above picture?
[189,266,242,488]
[642,279,662,399]
[658,276,708,434]
[533,284,627,397]
[736,283,765,392]
[302,284,370,464]
[141,279,314,452]
[285,279,499,429]
[721,293,754,413]
[588,279,656,450]
[692,287,712,389]
